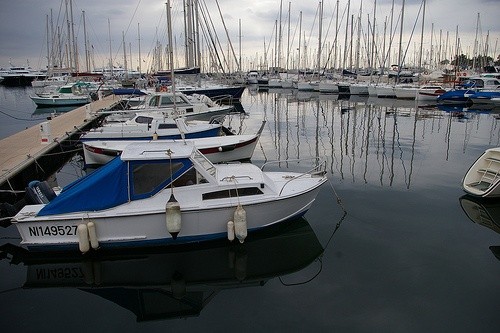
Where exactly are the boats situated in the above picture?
[462,146,500,201]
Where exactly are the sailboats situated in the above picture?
[0,1,500,258]
